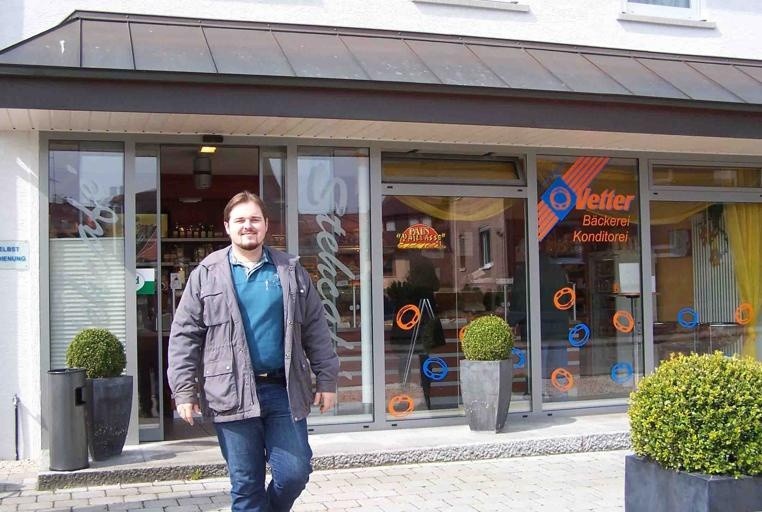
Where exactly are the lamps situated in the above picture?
[614,262,641,396]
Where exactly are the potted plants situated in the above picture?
[623,350,762,512]
[459,316,516,433]
[66,328,134,462]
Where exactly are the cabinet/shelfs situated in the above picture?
[136,237,360,337]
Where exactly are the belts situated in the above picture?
[256,373,286,387]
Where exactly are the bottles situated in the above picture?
[163,222,224,263]
[559,233,583,259]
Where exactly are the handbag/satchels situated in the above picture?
[421,317,445,350]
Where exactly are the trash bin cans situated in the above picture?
[47,368,90,471]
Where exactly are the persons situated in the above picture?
[507,239,573,401]
[166,190,341,511]
[386,248,446,411]
[481,292,520,339]
